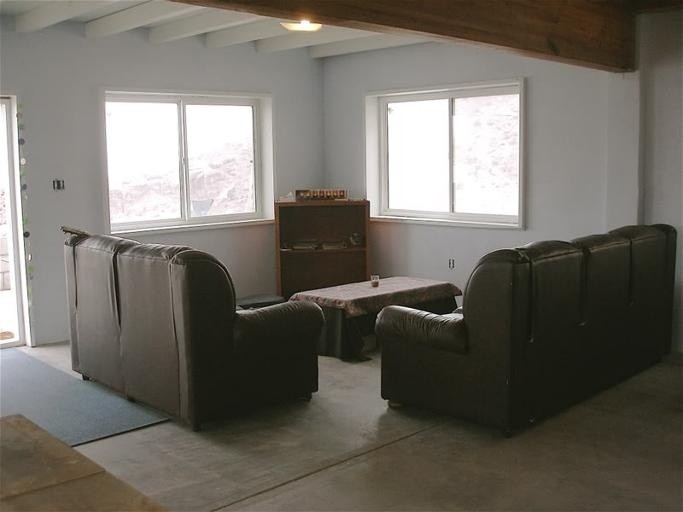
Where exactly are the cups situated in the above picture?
[370,274,379,287]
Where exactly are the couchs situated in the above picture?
[61,231,325,435]
[369,224,678,439]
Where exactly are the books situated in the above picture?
[292,239,348,250]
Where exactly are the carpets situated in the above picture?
[1,342,175,507]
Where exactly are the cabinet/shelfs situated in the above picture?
[278,204,370,304]
